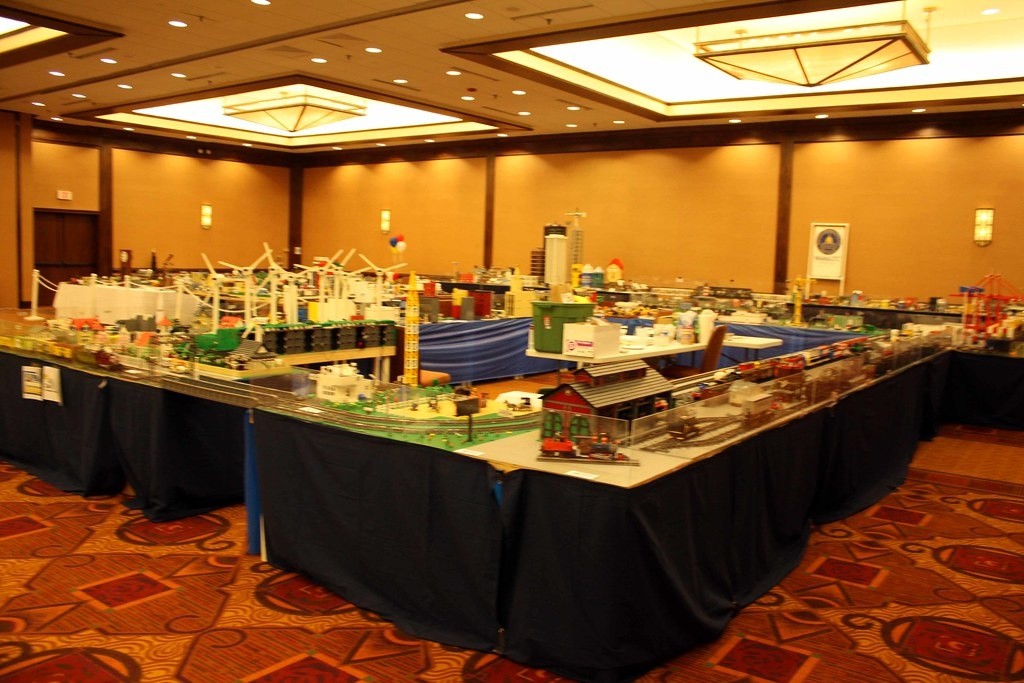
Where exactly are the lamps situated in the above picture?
[223,84,367,132]
[688,0,938,88]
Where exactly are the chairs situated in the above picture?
[661,323,727,377]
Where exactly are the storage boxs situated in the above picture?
[530,301,598,352]
[560,321,622,357]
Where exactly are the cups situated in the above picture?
[423,283,435,297]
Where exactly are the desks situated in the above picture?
[0,318,1024,683]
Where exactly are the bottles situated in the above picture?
[698,305,715,344]
[593,266,604,290]
[582,264,593,288]
